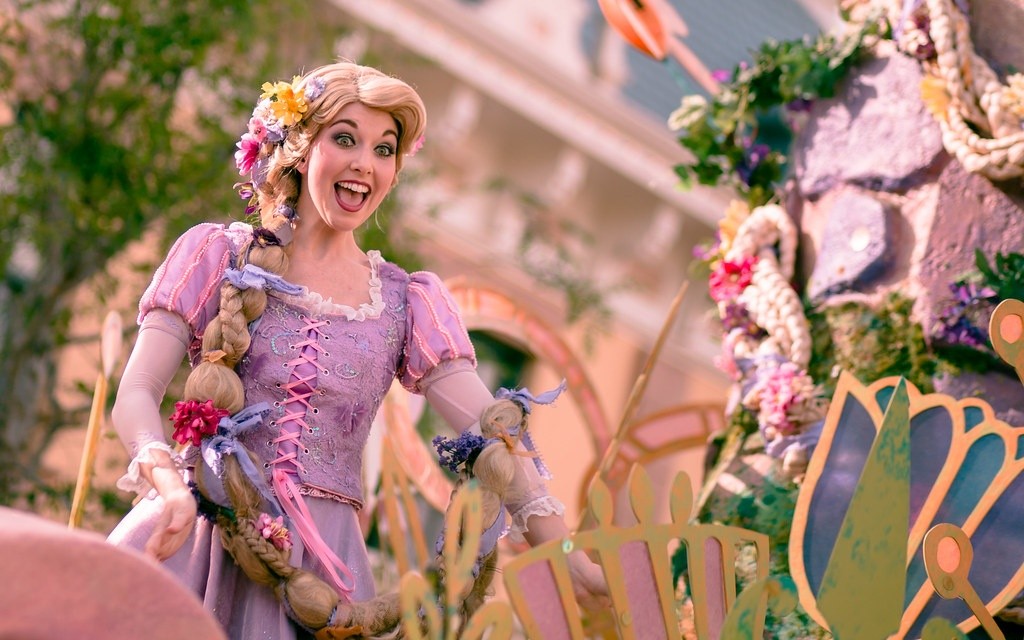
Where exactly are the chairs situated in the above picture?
[500,459,769,640]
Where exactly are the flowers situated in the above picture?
[230,75,309,216]
[167,399,231,447]
[665,17,1024,433]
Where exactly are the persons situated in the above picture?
[103,59,611,640]
